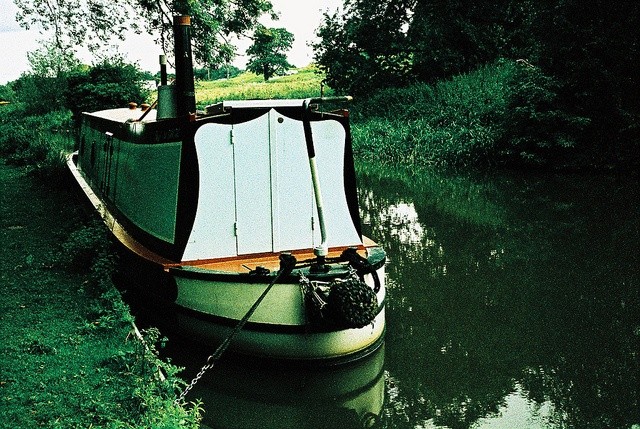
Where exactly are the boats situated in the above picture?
[62,15,387,361]
